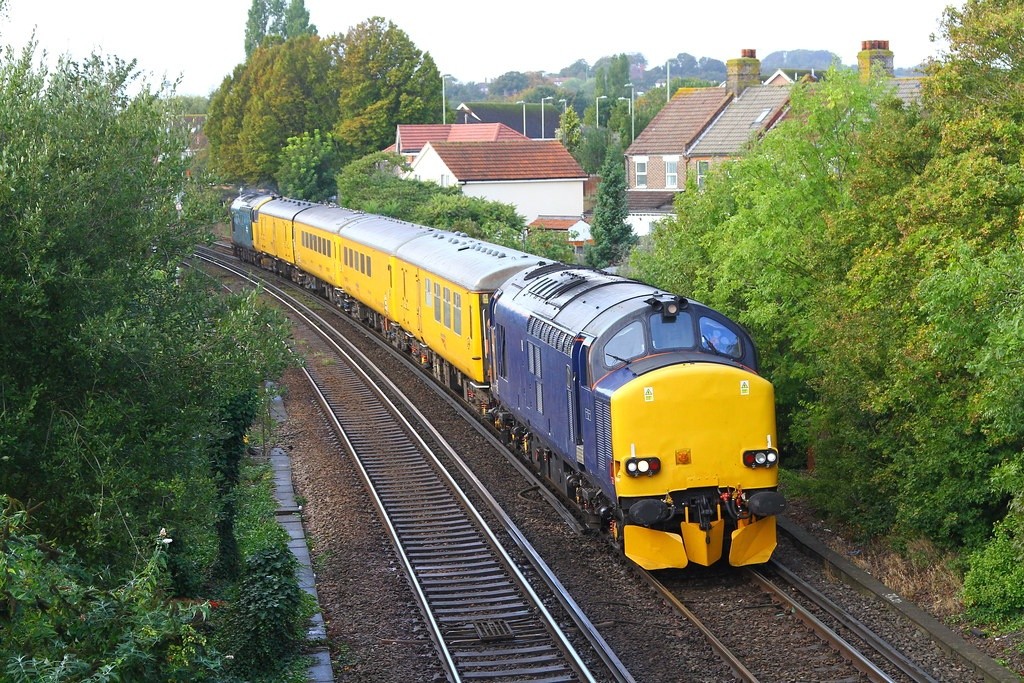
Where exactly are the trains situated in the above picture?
[229,188,788,571]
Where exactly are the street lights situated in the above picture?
[558,98,568,147]
[541,96,553,138]
[516,101,526,136]
[439,72,452,124]
[596,95,608,130]
[618,96,631,116]
[623,84,634,144]
